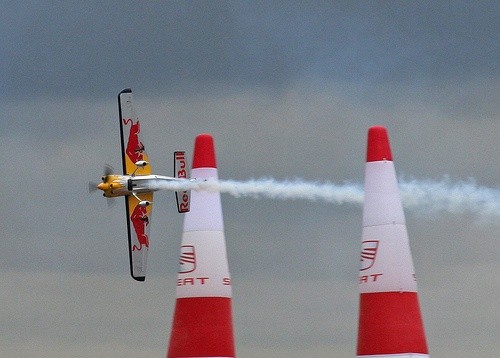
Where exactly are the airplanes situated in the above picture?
[96,85,195,285]
